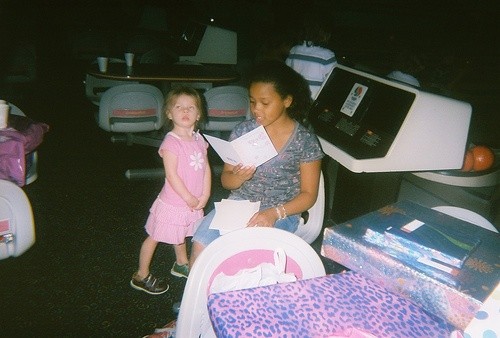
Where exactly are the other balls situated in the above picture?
[474,145,496,173]
[455,150,473,174]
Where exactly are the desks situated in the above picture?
[86,61,237,179]
[206,272,461,338]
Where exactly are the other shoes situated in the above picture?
[130,273,169,295]
[170,262,189,278]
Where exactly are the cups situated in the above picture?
[124,53,134,66]
[98,57,108,72]
[0,99,9,129]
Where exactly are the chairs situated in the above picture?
[294,169,325,243]
[94,83,164,133]
[174,227,327,338]
[86,57,139,106]
[0,178,36,260]
[201,85,250,131]
[157,60,213,98]
[9,101,37,184]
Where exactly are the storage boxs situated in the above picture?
[321,197,500,330]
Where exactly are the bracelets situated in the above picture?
[273,205,282,222]
[279,203,287,219]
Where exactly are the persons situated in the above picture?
[130,85,213,295]
[284,18,339,104]
[172,62,324,313]
